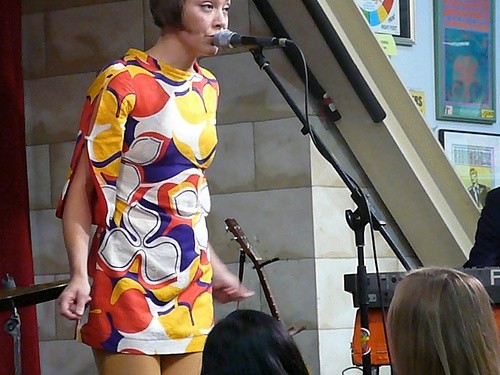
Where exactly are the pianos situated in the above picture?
[343,267,500,375]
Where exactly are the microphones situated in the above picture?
[213,29,295,47]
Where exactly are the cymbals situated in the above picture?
[0,278,72,314]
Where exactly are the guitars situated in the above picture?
[225,216,282,323]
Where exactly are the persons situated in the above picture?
[55,0,255,375]
[387,268,500,375]
[201,310,311,375]
[464,187,500,268]
